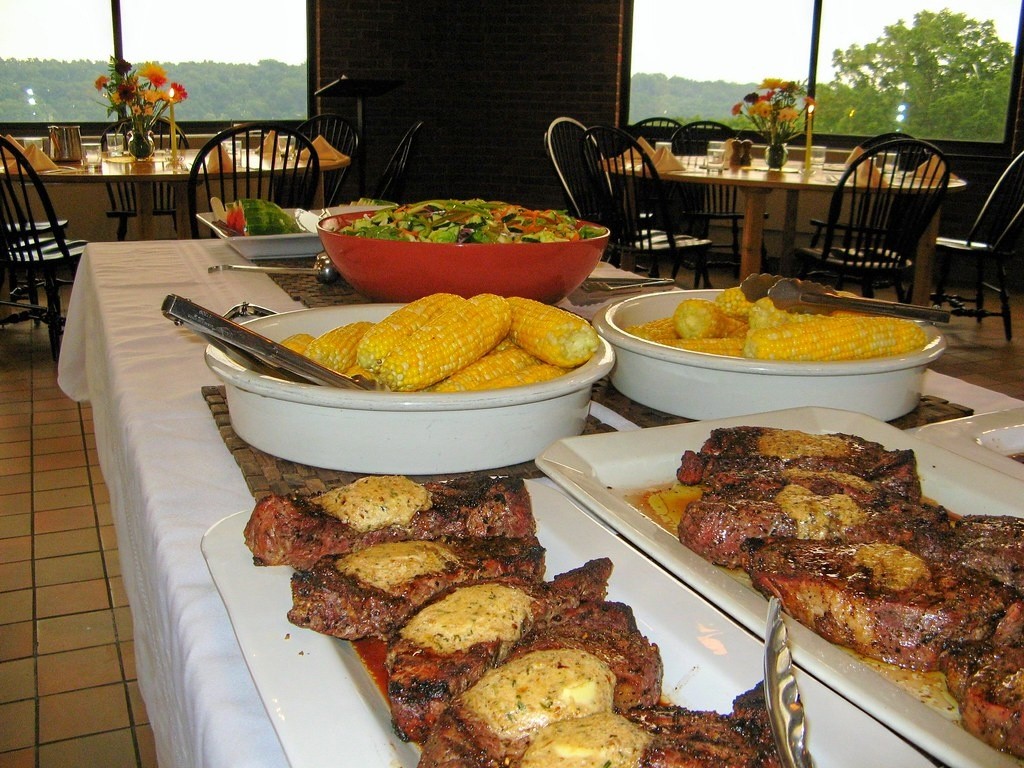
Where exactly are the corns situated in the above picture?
[275,290,601,392]
[622,285,930,363]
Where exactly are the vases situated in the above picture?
[128,118,154,159]
[765,142,788,168]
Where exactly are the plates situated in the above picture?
[901,407,1023,482]
[534,407,1024,768]
[196,208,324,260]
[299,206,394,234]
[201,477,936,768]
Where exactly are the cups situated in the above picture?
[655,141,672,152]
[153,150,165,160]
[278,136,296,160]
[877,152,900,174]
[106,134,123,156]
[42,138,50,158]
[811,145,827,166]
[869,157,876,166]
[221,141,242,167]
[709,140,726,163]
[81,143,102,167]
[707,149,726,172]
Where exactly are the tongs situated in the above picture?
[578,276,675,302]
[222,302,277,319]
[160,294,371,390]
[740,270,950,325]
[763,595,813,768]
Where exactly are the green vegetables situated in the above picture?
[338,200,607,243]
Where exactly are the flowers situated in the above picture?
[733,79,817,144]
[95,57,188,121]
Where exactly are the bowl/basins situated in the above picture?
[592,289,948,420]
[316,210,610,302]
[205,304,616,474]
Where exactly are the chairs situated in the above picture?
[543,116,769,290]
[375,119,425,199]
[295,113,361,206]
[186,122,321,239]
[101,115,190,241]
[0,133,94,359]
[794,133,1024,342]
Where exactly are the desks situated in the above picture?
[597,155,968,307]
[55,230,1024,768]
[0,137,351,238]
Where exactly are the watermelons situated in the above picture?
[224,197,304,236]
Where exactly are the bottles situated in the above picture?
[729,139,754,166]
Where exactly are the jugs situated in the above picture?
[48,126,82,161]
